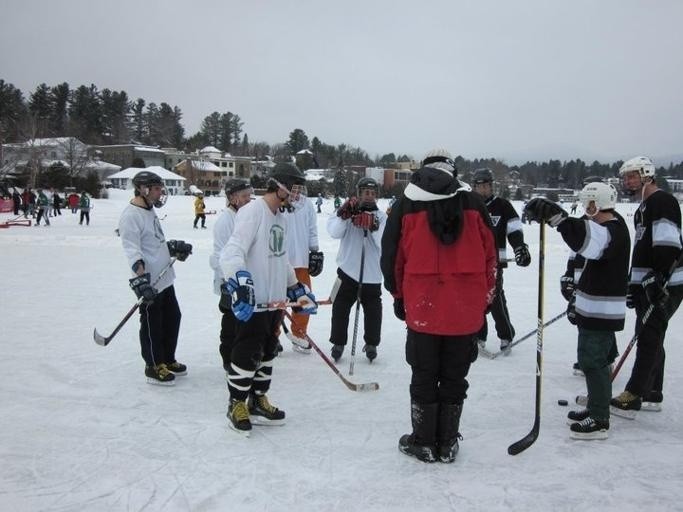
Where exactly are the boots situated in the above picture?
[438,400,465,464]
[145,362,176,382]
[398,398,438,464]
[246,393,286,421]
[227,399,252,431]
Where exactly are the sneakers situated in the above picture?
[569,413,611,433]
[330,344,344,359]
[611,390,642,412]
[366,344,378,359]
[291,334,313,349]
[500,338,511,351]
[567,399,590,421]
[165,359,187,373]
[642,390,664,403]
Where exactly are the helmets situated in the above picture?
[355,177,379,205]
[471,168,494,190]
[131,170,168,209]
[224,178,254,200]
[578,181,619,211]
[269,162,308,211]
[618,155,656,177]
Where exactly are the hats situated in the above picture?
[420,147,459,176]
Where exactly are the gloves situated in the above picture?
[393,298,406,320]
[641,269,672,309]
[218,283,231,314]
[559,259,578,301]
[307,251,324,277]
[350,209,377,229]
[166,239,192,262]
[512,241,532,267]
[226,270,256,323]
[521,195,571,228]
[127,272,159,305]
[565,296,578,326]
[336,196,361,220]
[626,276,636,310]
[286,280,318,315]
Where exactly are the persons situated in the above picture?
[210,178,253,377]
[334,194,342,212]
[325,178,387,361]
[226,164,306,432]
[9,184,91,226]
[194,192,208,230]
[469,167,531,354]
[382,148,500,463]
[388,195,399,207]
[274,196,324,351]
[315,193,326,214]
[118,171,193,382]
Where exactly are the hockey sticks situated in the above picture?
[577,260,677,405]
[94,258,177,346]
[254,278,342,310]
[480,313,569,359]
[508,220,545,455]
[285,312,380,392]
